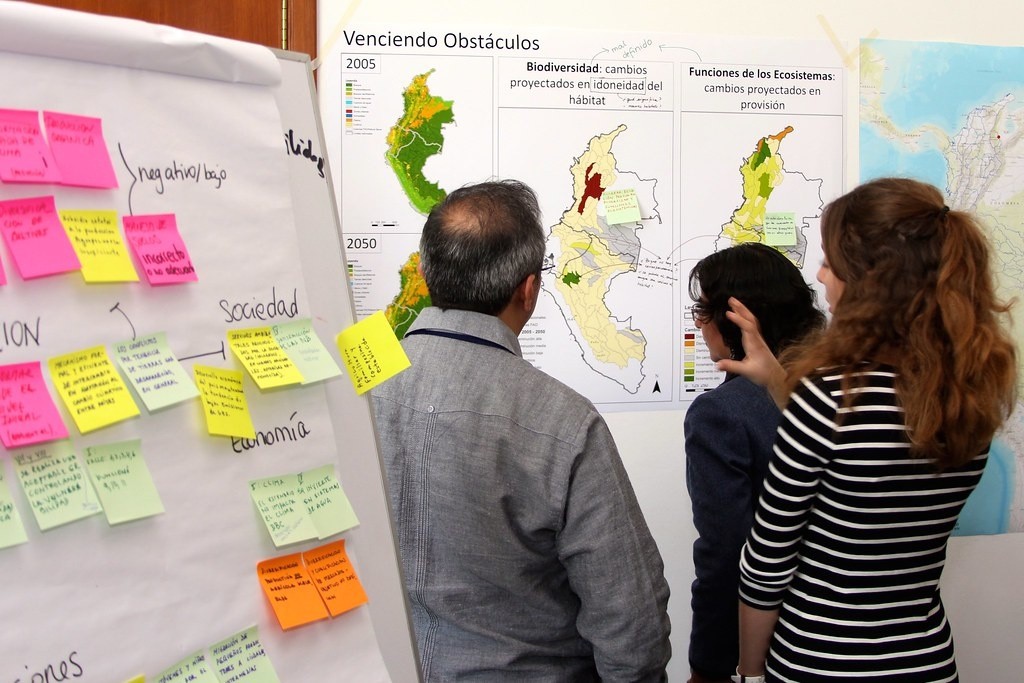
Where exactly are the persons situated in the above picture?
[369,180,672,683]
[717,179,1018,683]
[684,242,827,683]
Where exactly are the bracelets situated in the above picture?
[731,665,765,683]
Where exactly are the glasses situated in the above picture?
[691,303,715,322]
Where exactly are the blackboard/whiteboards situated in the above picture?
[0,0,426,683]
